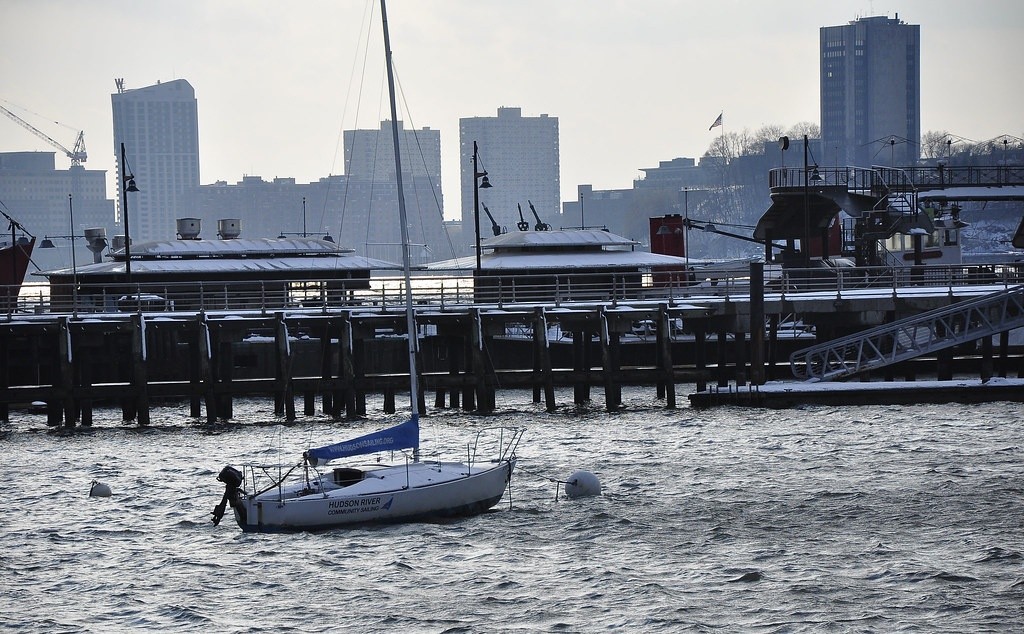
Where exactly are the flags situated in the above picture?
[708,112,723,131]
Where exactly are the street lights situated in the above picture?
[68,194,78,313]
[119,142,140,292]
[804,134,822,268]
[469,140,494,304]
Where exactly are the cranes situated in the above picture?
[0,99,89,167]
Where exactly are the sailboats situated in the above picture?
[203,0,529,533]
